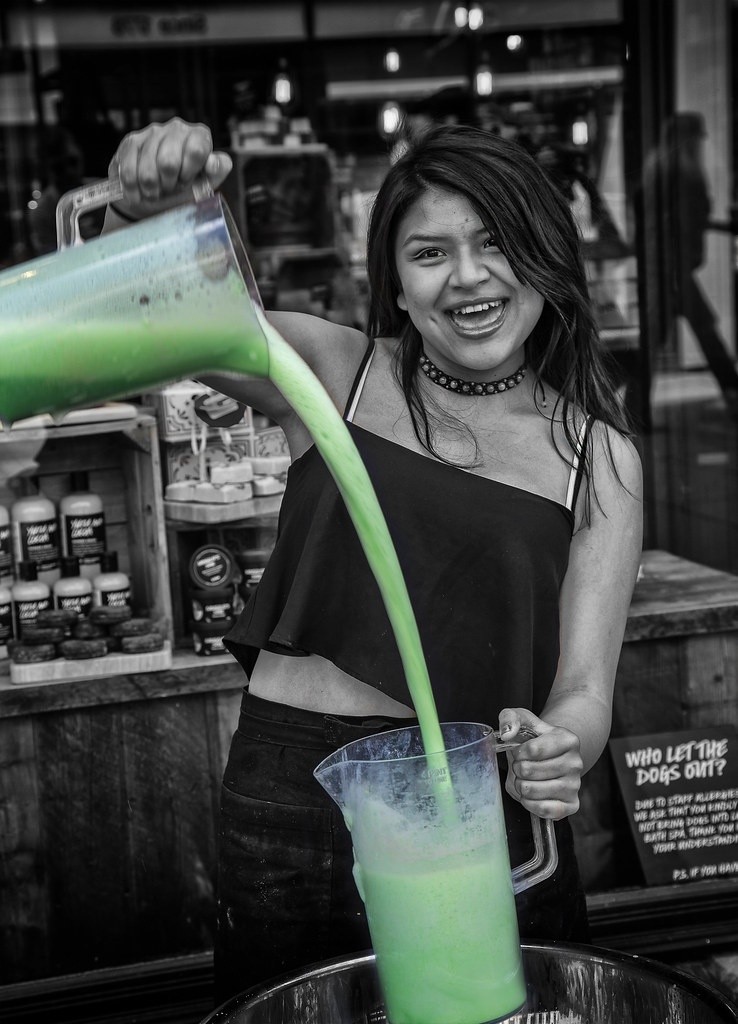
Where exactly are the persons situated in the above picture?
[94,118,645,1003]
[624,113,738,435]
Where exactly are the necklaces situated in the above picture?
[418,353,528,396]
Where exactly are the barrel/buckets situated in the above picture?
[197,938,738,1024]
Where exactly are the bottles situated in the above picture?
[0,467,133,667]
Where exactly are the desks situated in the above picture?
[0,550,738,1024]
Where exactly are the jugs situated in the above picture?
[312,721,559,1024]
[0,165,270,428]
